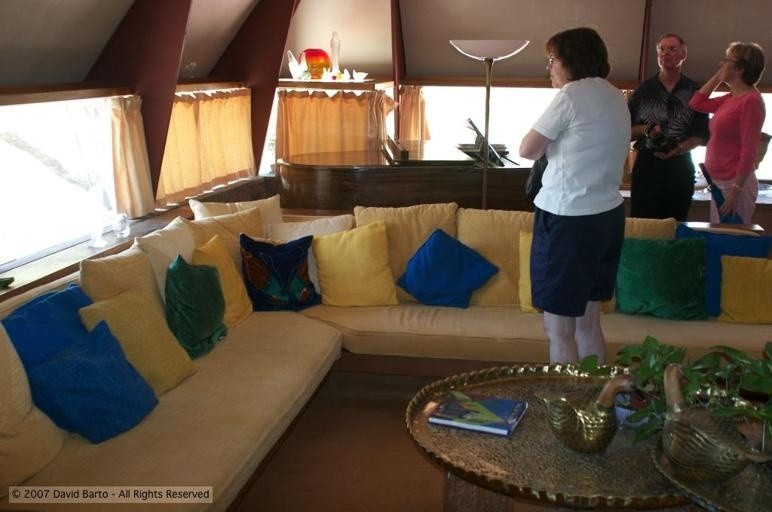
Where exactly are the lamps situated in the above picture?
[448,38,531,209]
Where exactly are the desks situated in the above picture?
[618,188,772,236]
[276,150,535,211]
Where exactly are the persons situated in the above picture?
[518,27,632,367]
[687,42,766,226]
[627,35,709,222]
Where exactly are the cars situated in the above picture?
[112,207,131,240]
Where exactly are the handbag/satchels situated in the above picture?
[644,123,654,137]
[733,184,743,192]
[677,143,685,156]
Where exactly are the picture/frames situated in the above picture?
[276,150,535,211]
[618,188,772,236]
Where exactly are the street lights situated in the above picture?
[754,133,772,169]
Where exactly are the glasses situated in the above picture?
[448,38,531,209]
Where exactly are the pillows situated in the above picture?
[0,191,354,498]
[352,197,772,328]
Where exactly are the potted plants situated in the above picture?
[572,334,772,447]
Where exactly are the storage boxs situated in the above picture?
[0,268,342,510]
[283,214,772,381]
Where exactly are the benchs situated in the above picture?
[283,214,772,381]
[0,268,342,510]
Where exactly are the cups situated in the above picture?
[723,58,738,64]
[658,45,679,53]
[548,56,561,65]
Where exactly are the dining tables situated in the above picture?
[572,334,772,447]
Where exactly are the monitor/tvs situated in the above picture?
[458,143,507,154]
[427,389,528,437]
